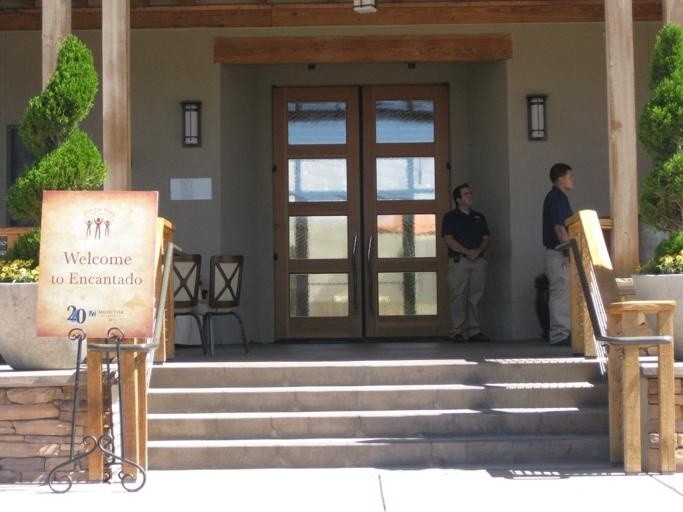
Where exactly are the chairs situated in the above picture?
[194,255,249,357]
[172,254,207,355]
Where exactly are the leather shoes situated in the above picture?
[454,332,491,344]
[551,339,571,347]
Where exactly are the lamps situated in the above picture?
[181,101,202,148]
[526,94,548,142]
[353,0,378,16]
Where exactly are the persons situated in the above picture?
[440,182,491,343]
[542,163,576,346]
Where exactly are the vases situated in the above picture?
[0,283,88,371]
[630,273,683,361]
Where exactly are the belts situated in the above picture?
[545,245,558,250]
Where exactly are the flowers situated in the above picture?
[0,258,40,284]
[634,250,683,274]
